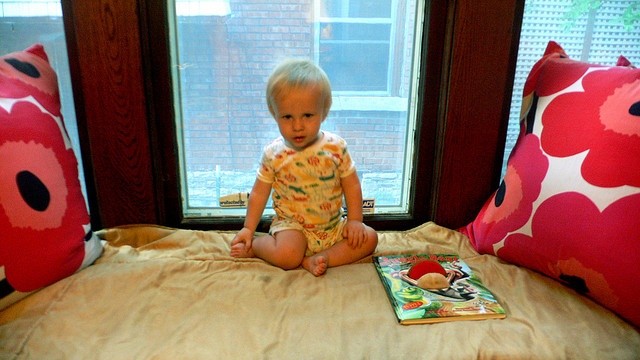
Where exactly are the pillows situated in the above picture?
[0,43,105,313]
[459,41,640,327]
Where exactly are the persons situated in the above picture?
[230,59,378,277]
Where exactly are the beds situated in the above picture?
[1,222,639,360]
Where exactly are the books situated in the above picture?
[372,253,506,325]
